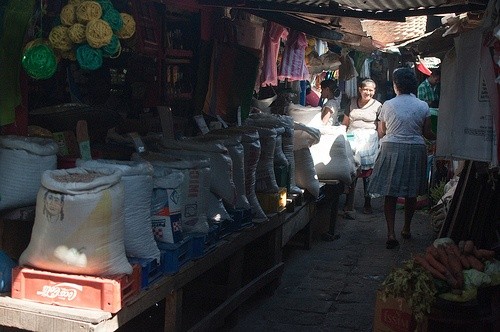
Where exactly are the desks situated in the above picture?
[0,180,345,332]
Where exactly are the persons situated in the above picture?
[299,80,341,127]
[367,67,432,252]
[417,70,440,108]
[341,79,387,220]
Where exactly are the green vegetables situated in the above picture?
[422,178,446,213]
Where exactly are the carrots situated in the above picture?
[415,240,492,290]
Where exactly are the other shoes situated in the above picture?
[401,231,410,240]
[363,208,372,214]
[345,211,356,219]
[386,240,398,249]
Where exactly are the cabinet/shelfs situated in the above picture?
[132,13,198,112]
